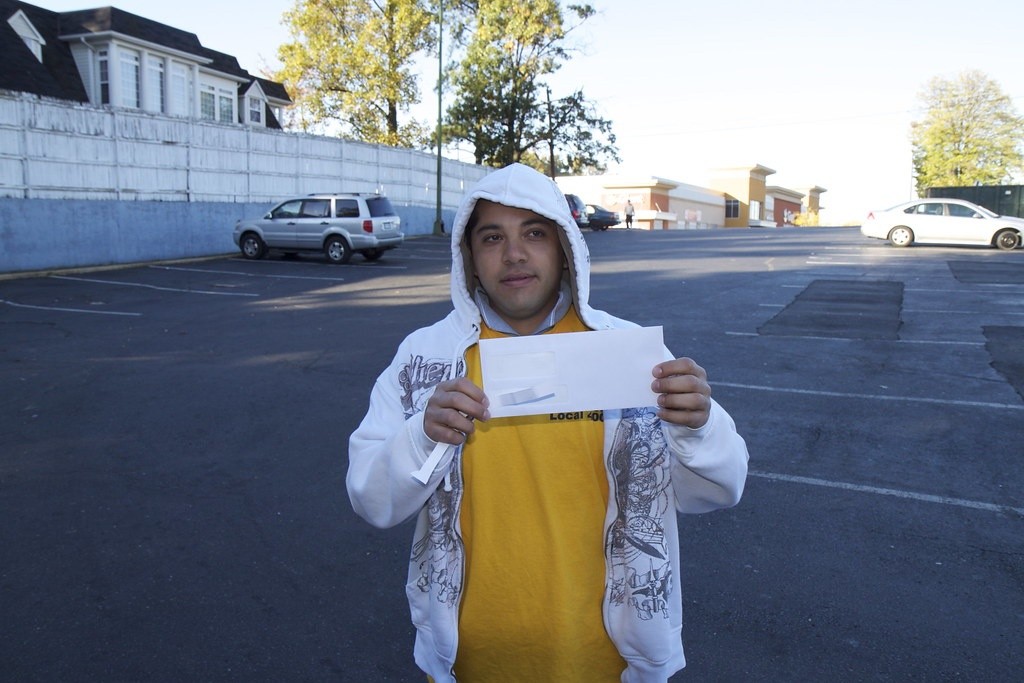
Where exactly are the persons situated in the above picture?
[347,163,749,683]
[624,200,634,227]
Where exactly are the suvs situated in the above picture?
[232,191,406,264]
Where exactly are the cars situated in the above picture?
[859,196,1024,253]
[585,204,621,231]
[564,193,591,228]
